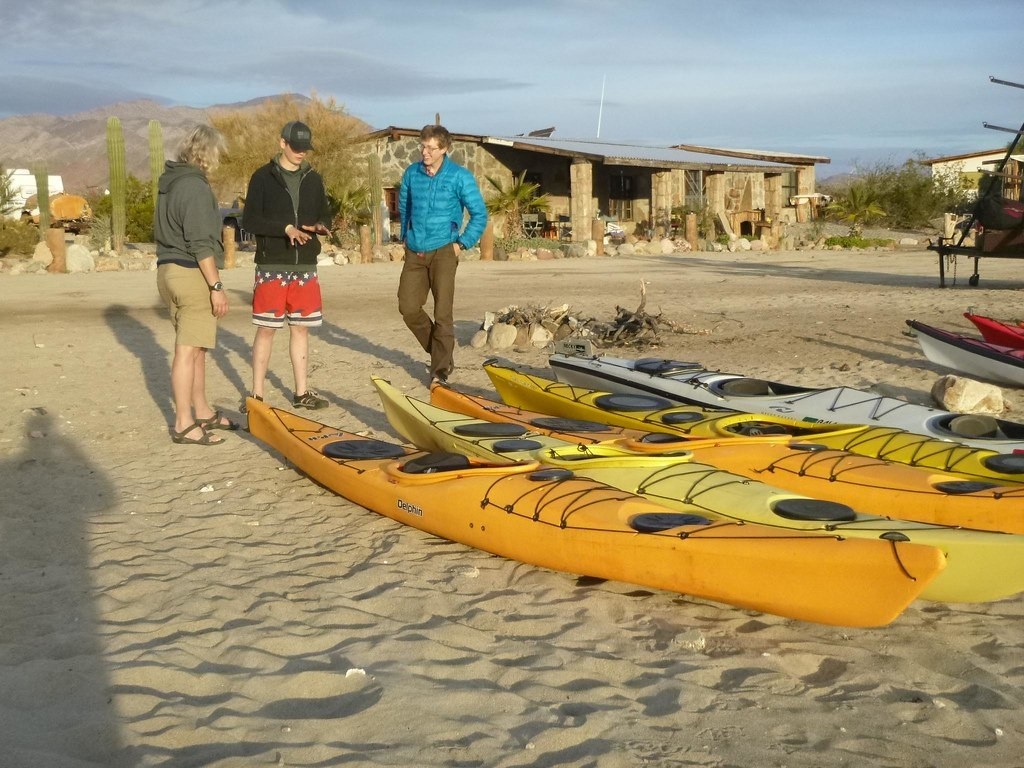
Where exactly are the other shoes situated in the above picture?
[429,377,451,389]
[448,340,455,375]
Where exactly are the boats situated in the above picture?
[481,357,1024,483]
[965,311,1024,350]
[907,318,1024,386]
[372,374,1023,603]
[427,376,1024,535]
[242,396,949,627]
[548,338,1024,456]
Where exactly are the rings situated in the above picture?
[323,226,324,228]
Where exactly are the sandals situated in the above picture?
[173,423,225,445]
[293,390,329,410]
[195,411,239,430]
[240,394,263,415]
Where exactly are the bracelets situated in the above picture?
[285,225,293,233]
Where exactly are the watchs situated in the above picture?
[209,282,223,291]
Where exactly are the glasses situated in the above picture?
[420,145,440,153]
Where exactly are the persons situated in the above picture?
[398,126,487,389]
[155,125,241,444]
[238,121,331,412]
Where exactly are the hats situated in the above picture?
[281,121,315,150]
